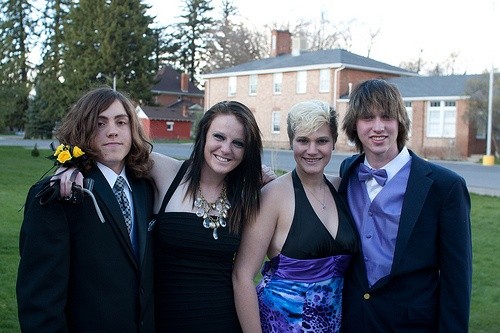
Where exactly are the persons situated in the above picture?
[233,100,364,333]
[15,88,157,333]
[51,100,280,333]
[322,79,472,333]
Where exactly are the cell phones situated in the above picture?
[83,178,95,198]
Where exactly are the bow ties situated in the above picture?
[358,163,388,187]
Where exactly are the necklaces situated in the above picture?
[302,179,325,209]
[193,182,231,240]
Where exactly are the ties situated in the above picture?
[114,177,132,236]
